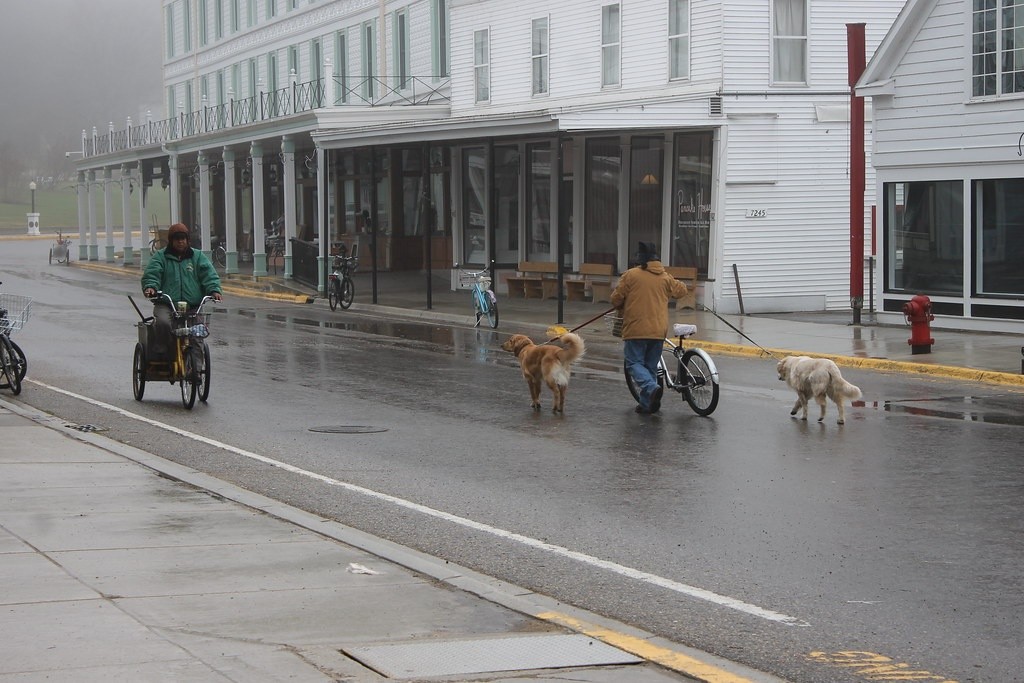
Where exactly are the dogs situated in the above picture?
[501,333,589,413]
[777,356,863,424]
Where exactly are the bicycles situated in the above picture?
[603,312,720,417]
[210,241,227,268]
[327,254,357,311]
[454,260,499,329]
[0,280,34,395]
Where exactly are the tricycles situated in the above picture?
[49,230,71,267]
[132,290,220,410]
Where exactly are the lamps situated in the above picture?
[640,172,659,185]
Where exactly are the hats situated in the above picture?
[168,223,190,246]
[637,239,658,269]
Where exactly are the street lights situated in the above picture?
[29,181,36,212]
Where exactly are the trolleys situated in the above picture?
[149,213,170,256]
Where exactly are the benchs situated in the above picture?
[565,263,614,306]
[669,267,698,310]
[506,260,558,300]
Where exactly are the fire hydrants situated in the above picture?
[902,292,935,355]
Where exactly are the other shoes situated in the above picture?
[648,385,663,413]
[635,404,646,413]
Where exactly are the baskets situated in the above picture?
[0,293,34,333]
[171,310,211,338]
[460,271,491,289]
[603,312,624,337]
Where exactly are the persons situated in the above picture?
[610,241,688,415]
[140,224,224,384]
